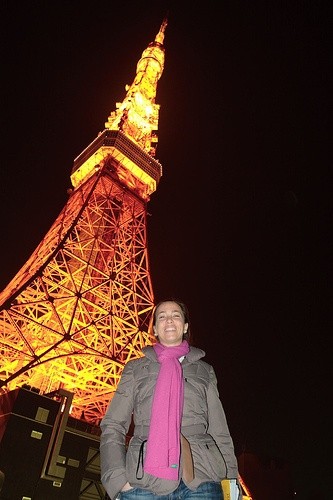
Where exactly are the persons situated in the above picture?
[100,297,242,500]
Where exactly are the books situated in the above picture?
[220,478,240,500]
